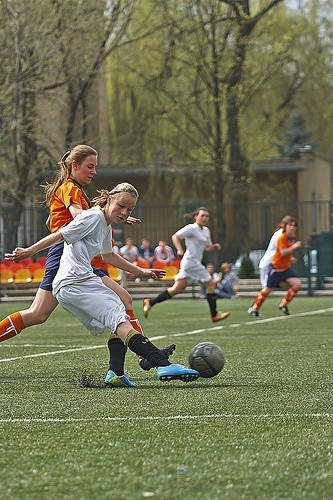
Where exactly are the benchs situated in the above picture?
[0,276,333,295]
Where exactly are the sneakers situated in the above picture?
[278,304,290,316]
[247,308,263,319]
[104,370,136,387]
[156,364,201,382]
[138,344,176,370]
[143,298,150,319]
[211,312,230,323]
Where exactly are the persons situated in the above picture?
[0,145,176,371]
[154,241,175,264]
[200,263,219,299]
[119,238,139,264]
[137,238,156,268]
[247,220,302,317]
[112,238,122,256]
[214,262,241,299]
[259,215,294,289]
[4,183,200,386]
[143,206,231,323]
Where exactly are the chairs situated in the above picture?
[0,257,181,281]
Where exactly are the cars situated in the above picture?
[231,249,267,278]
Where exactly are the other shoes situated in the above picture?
[135,277,141,283]
[147,278,154,283]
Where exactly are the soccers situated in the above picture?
[190,342,226,378]
